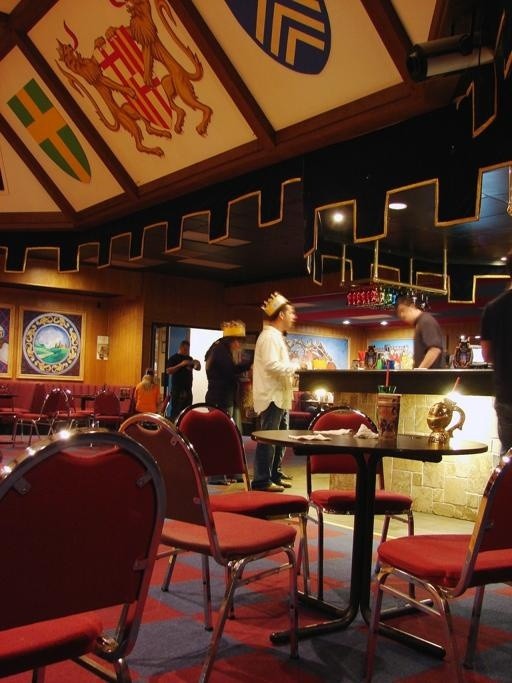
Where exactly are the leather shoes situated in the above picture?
[280,471,294,479]
[252,483,284,492]
[277,479,292,487]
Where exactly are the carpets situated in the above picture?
[0,431,512,681]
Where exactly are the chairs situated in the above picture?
[1,423,169,682]
[359,444,512,682]
[158,400,314,620]
[0,374,134,449]
[294,403,420,605]
[116,409,303,683]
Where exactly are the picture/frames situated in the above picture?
[15,303,88,383]
[0,301,17,381]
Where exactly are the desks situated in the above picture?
[247,424,492,660]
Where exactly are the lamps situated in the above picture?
[400,0,499,83]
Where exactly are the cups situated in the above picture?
[376,392,402,436]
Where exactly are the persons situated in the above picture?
[393,295,445,369]
[164,340,203,421]
[479,247,512,466]
[134,368,161,414]
[249,291,315,494]
[203,320,253,487]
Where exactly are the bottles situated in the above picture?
[355,345,410,367]
[345,286,431,311]
